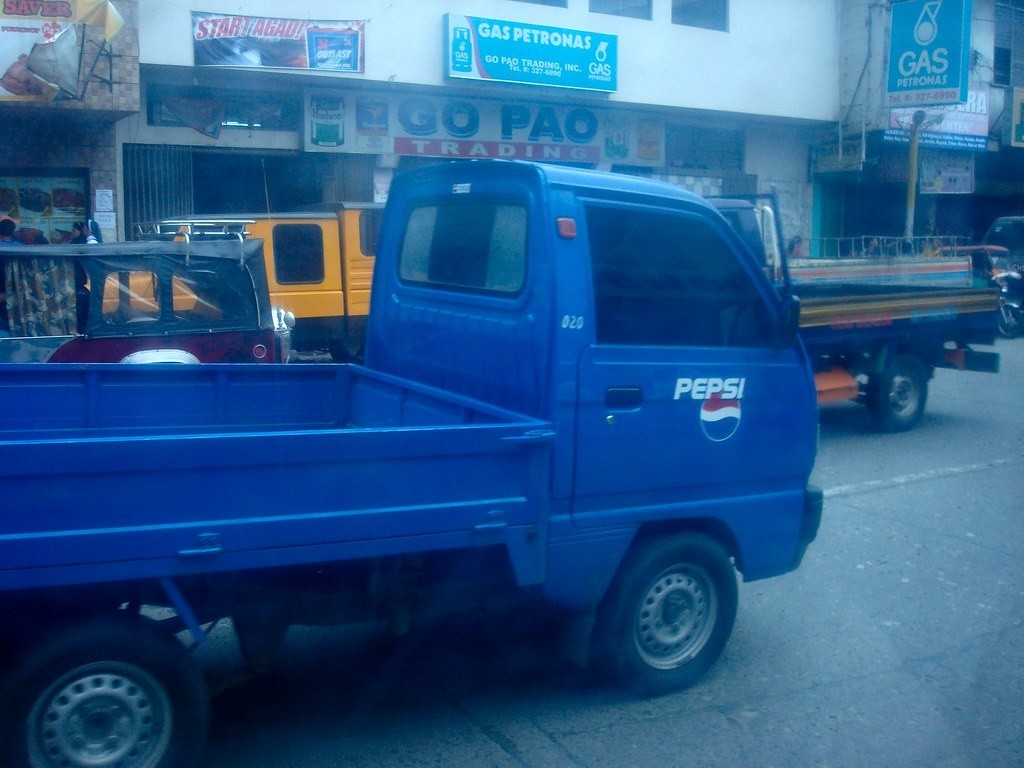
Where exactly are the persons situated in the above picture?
[861,235,941,258]
[0,219,103,246]
[786,235,804,257]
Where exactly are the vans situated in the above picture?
[983,215,1024,278]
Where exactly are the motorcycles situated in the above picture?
[936,242,1021,339]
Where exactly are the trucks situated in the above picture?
[76,202,391,368]
[0,156,820,765]
[660,183,1003,433]
